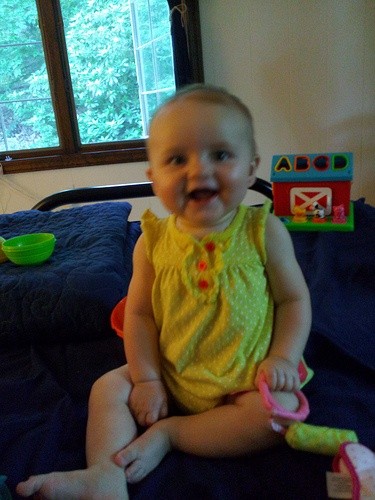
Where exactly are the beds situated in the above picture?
[0,180,375,500]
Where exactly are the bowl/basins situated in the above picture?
[1,233,56,266]
[110,296,126,340]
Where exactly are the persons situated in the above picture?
[13,81,315,500]
